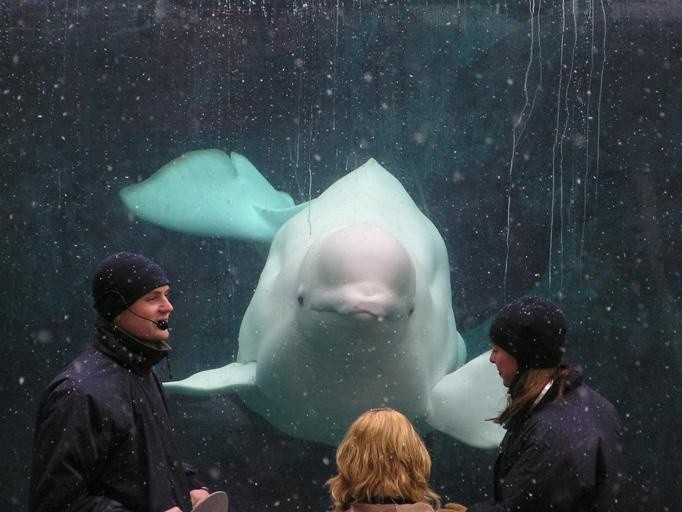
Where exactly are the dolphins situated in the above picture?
[117,146,514,451]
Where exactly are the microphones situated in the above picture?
[125,306,167,330]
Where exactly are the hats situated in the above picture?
[489,294,568,370]
[91,251,172,325]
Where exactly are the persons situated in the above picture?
[334,407,440,512]
[440,294,624,512]
[29,251,210,512]
